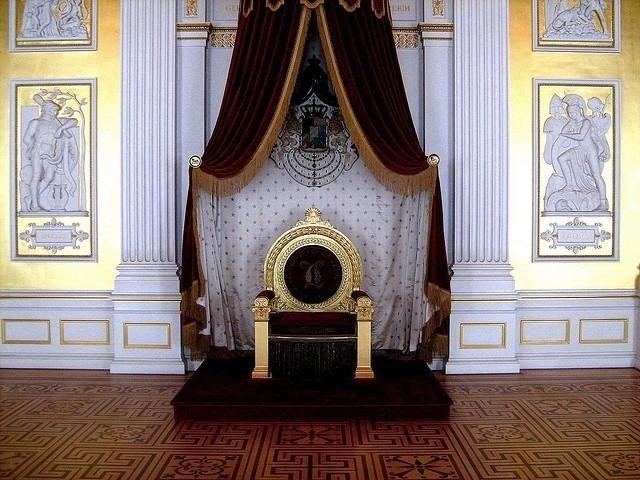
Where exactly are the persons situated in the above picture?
[21,95,76,218]
[540,88,613,213]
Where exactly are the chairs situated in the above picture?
[250,205,379,385]
